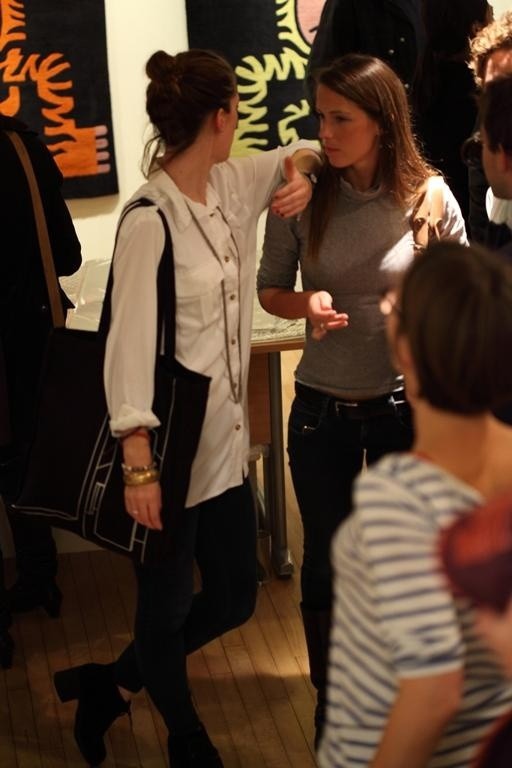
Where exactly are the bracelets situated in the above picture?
[119,461,161,486]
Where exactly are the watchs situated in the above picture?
[301,171,319,186]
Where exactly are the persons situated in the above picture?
[450,12,512,251]
[477,70,512,263]
[304,1,496,216]
[0,112,84,669]
[255,50,472,754]
[315,237,512,768]
[51,44,324,768]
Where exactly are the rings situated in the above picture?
[132,510,138,515]
[319,323,325,332]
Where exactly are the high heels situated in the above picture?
[167,724,226,765]
[6,580,64,618]
[55,663,130,765]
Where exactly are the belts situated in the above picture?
[294,381,411,422]
[512,663,512,672]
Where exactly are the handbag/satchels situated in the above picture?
[9,199,211,565]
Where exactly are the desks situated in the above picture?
[248,334,307,579]
